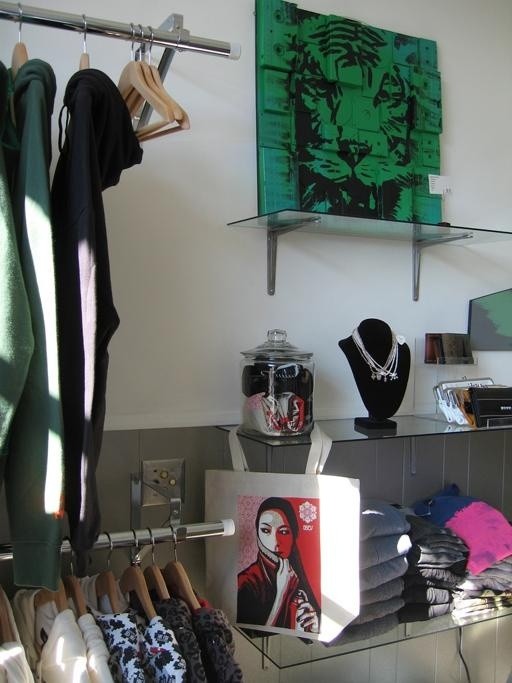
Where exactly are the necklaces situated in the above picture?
[350,324,402,382]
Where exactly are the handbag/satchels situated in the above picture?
[432,377,512,427]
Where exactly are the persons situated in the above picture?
[238,496,320,632]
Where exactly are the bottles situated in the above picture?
[238,325,318,439]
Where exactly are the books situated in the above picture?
[423,331,475,363]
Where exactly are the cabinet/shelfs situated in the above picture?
[0,520,237,666]
[0,0,194,143]
[215,209,512,668]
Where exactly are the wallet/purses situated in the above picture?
[424,333,475,364]
